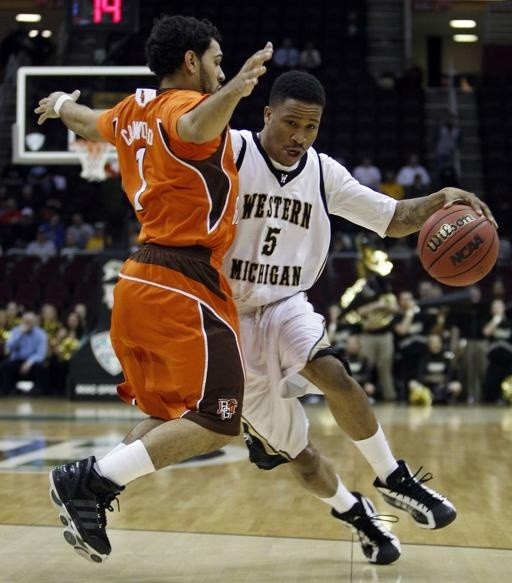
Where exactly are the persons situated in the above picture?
[230,70,499,564]
[274,39,322,68]
[308,111,512,407]
[1,74,103,399]
[33,16,273,563]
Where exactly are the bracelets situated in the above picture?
[53,94,73,117]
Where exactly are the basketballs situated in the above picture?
[416,202,500,286]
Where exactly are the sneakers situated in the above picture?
[49,456,125,564]
[331,491,402,565]
[373,459,457,530]
